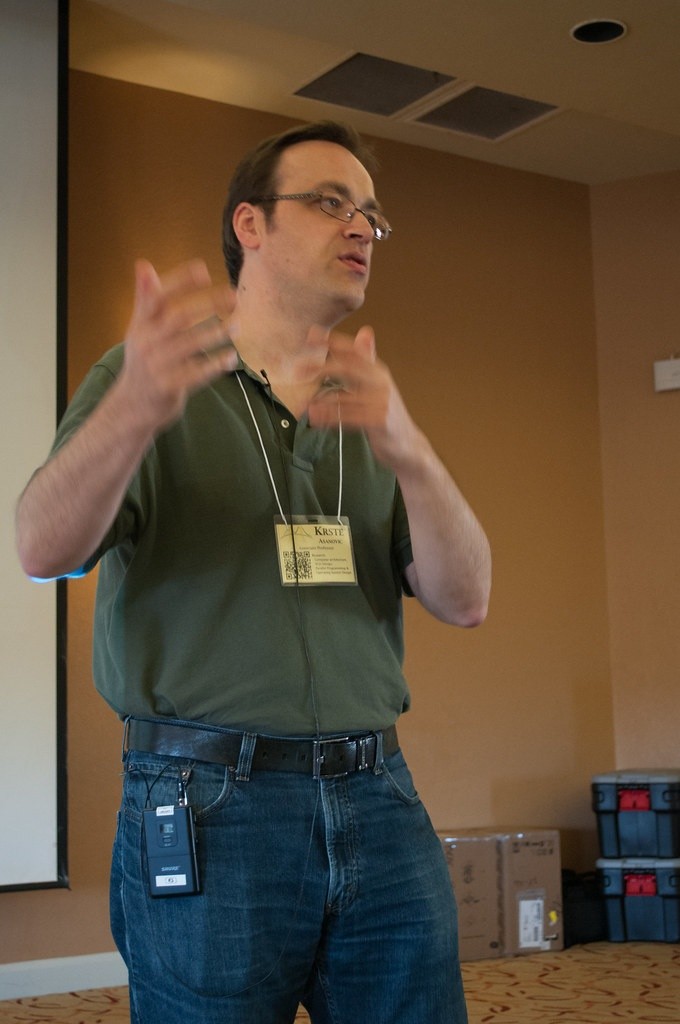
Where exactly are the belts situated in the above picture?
[127,719,400,778]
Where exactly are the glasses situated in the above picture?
[249,192,392,240]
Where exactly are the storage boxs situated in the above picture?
[427,767,680,962]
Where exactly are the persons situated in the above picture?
[16,119,491,1024]
